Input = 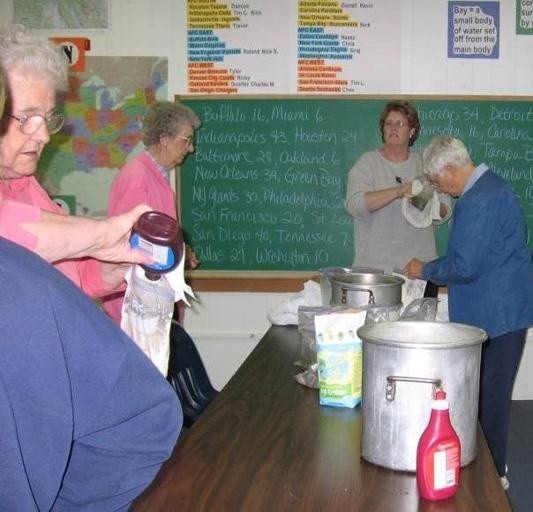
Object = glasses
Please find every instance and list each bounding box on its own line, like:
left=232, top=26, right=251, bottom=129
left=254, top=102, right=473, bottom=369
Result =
left=383, top=117, right=420, bottom=133
left=173, top=133, right=194, bottom=148
left=3, top=104, right=66, bottom=138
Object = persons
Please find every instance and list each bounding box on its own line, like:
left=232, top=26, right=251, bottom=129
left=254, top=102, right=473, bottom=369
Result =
left=0, top=61, right=183, bottom=512
left=343, top=101, right=452, bottom=298
left=107, top=99, right=201, bottom=323
left=0, top=19, right=156, bottom=299
left=404, top=134, right=533, bottom=492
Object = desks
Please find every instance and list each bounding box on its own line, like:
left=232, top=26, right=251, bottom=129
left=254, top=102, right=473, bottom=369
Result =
left=129, top=323, right=516, bottom=512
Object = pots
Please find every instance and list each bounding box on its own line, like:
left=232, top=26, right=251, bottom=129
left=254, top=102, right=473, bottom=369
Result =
left=355, top=321, right=487, bottom=473
left=315, top=266, right=405, bottom=307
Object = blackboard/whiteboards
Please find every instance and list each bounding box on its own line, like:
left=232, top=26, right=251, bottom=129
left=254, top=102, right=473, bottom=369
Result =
left=175, top=95, right=533, bottom=280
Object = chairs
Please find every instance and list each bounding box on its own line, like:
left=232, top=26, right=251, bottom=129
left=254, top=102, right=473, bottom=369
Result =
left=166, top=320, right=218, bottom=423
